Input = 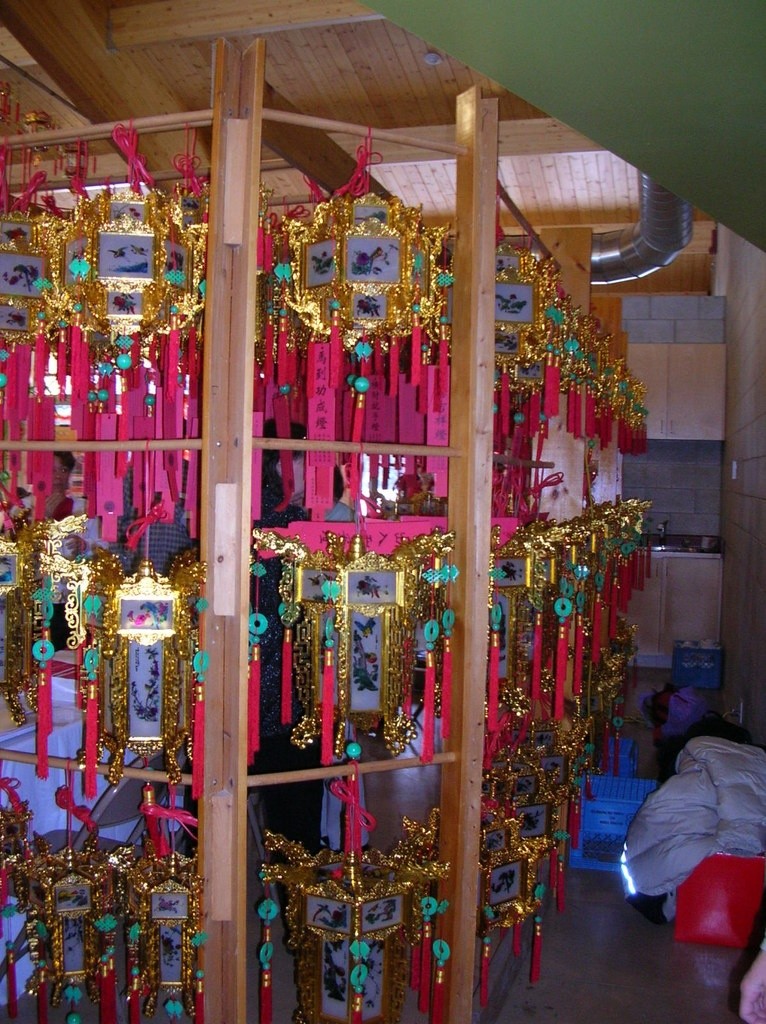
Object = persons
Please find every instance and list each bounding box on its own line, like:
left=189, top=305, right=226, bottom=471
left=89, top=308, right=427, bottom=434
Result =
left=8, top=417, right=370, bottom=955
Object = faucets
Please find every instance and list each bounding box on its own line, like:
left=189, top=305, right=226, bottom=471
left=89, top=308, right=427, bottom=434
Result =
left=656, top=520, right=668, bottom=539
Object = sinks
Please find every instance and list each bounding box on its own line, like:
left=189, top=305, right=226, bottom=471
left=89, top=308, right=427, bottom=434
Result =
left=650, top=533, right=723, bottom=553
